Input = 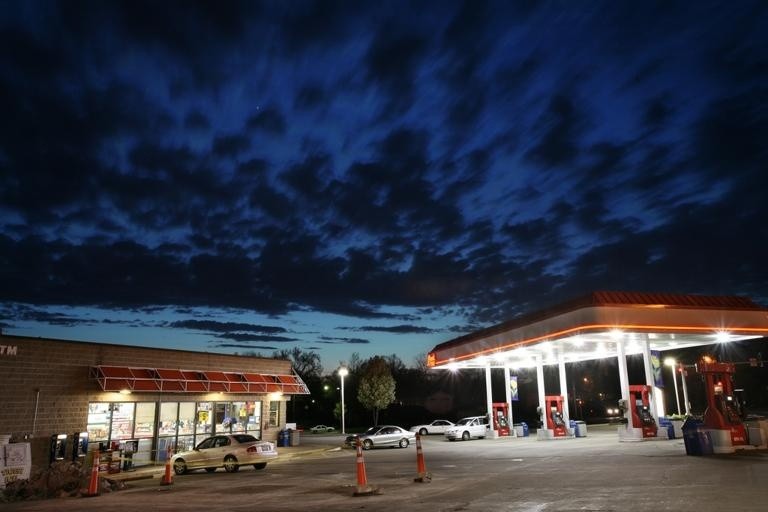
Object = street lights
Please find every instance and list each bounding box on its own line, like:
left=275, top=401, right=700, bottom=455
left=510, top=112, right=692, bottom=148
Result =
left=661, top=356, right=681, bottom=415
left=571, top=375, right=592, bottom=420
left=337, top=367, right=348, bottom=434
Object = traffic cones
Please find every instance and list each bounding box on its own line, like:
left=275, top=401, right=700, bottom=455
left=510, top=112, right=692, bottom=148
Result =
left=347, top=442, right=377, bottom=497
left=411, top=432, right=430, bottom=482
left=81, top=449, right=104, bottom=497
left=159, top=444, right=175, bottom=486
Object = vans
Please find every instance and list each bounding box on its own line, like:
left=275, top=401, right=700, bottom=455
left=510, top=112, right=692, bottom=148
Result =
left=443, top=416, right=489, bottom=443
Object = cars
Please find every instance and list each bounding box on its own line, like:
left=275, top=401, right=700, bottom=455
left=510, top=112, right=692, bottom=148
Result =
left=409, top=419, right=456, bottom=437
left=603, top=406, right=619, bottom=417
left=310, top=425, right=336, bottom=435
left=296, top=426, right=305, bottom=432
left=169, top=433, right=278, bottom=477
left=344, top=424, right=417, bottom=451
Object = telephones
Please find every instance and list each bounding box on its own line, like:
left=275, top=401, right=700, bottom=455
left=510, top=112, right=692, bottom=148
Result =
left=55, top=441, right=62, bottom=450
left=78, top=439, right=84, bottom=449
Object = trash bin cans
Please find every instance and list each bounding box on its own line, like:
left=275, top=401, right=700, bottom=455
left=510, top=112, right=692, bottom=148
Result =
left=681, top=417, right=714, bottom=456
left=278, top=430, right=299, bottom=447
left=513, top=422, right=528, bottom=437
left=659, top=417, right=685, bottom=440
left=744, top=417, right=768, bottom=445
left=569, top=420, right=587, bottom=438
